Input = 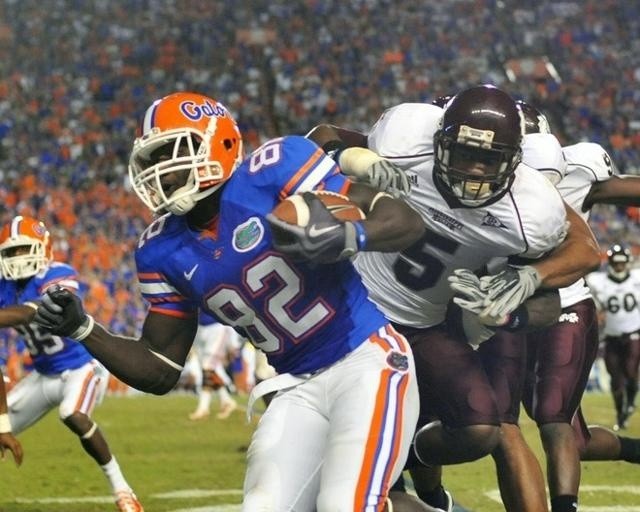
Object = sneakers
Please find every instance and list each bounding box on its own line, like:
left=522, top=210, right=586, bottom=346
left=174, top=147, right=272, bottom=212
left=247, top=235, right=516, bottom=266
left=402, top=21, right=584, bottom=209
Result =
left=187, top=408, right=209, bottom=421
left=113, top=488, right=145, bottom=511
left=217, top=399, right=236, bottom=419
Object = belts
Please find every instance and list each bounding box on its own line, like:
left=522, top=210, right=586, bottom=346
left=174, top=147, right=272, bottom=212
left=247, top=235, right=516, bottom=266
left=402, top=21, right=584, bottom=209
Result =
left=246, top=358, right=315, bottom=425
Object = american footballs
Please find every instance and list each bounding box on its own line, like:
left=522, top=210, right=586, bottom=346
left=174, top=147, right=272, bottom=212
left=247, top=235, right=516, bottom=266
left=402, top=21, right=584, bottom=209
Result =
left=272, top=190, right=365, bottom=228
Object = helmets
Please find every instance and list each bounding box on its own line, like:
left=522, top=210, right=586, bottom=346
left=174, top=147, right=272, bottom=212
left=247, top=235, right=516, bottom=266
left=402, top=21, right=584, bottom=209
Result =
left=605, top=243, right=634, bottom=282
left=127, top=92, right=242, bottom=219
left=1, top=215, right=53, bottom=283
left=431, top=83, right=524, bottom=210
left=518, top=98, right=553, bottom=137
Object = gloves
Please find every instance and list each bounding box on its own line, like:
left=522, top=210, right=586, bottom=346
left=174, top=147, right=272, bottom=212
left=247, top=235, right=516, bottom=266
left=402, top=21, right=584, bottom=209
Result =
left=263, top=192, right=360, bottom=268
left=32, top=284, right=96, bottom=344
left=449, top=267, right=508, bottom=328
left=477, top=264, right=543, bottom=319
left=367, top=159, right=412, bottom=199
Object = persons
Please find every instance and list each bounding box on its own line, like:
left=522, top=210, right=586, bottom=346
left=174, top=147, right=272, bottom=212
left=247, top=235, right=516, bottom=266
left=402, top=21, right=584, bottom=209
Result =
left=0, top=0, right=639, bottom=511
